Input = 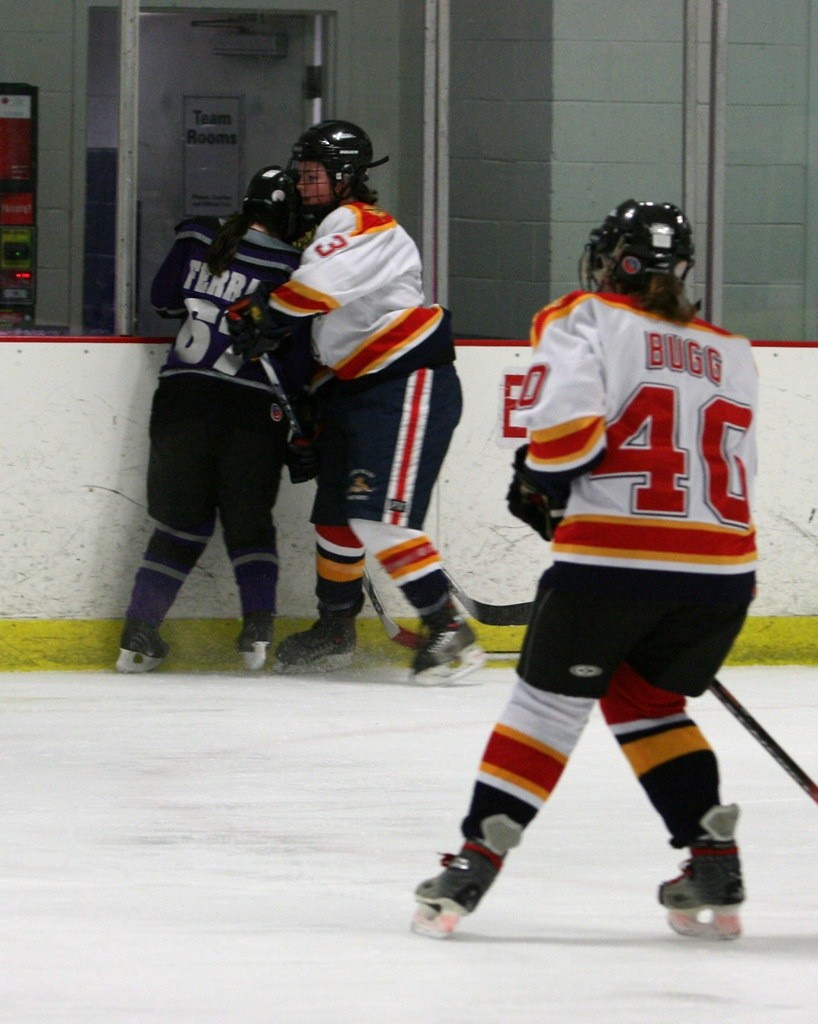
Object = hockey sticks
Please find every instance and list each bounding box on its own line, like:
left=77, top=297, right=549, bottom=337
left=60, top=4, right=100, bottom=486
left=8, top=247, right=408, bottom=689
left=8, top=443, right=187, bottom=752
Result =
left=436, top=561, right=536, bottom=627
left=261, top=350, right=435, bottom=651
left=554, top=517, right=818, bottom=805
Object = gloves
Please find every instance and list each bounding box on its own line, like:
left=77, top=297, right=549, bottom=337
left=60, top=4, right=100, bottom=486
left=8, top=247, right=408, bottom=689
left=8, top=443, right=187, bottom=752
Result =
left=283, top=417, right=332, bottom=484
left=223, top=292, right=286, bottom=361
left=504, top=443, right=575, bottom=541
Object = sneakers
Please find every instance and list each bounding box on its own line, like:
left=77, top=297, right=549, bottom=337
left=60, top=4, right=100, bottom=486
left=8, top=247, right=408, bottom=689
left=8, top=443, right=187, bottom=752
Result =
left=657, top=803, right=746, bottom=940
left=408, top=598, right=484, bottom=687
left=271, top=592, right=366, bottom=675
left=235, top=609, right=276, bottom=671
left=115, top=615, right=170, bottom=673
left=409, top=815, right=521, bottom=938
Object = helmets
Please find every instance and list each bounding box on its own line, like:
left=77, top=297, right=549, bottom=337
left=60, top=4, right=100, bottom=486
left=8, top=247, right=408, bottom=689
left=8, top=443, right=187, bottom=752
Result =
left=577, top=199, right=697, bottom=300
left=242, top=164, right=318, bottom=251
left=283, top=118, right=374, bottom=218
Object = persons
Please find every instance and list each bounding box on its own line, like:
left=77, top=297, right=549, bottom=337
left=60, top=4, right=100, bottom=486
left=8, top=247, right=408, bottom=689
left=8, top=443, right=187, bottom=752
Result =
left=409, top=200, right=760, bottom=940
left=115, top=165, right=313, bottom=674
left=224, top=119, right=486, bottom=685
